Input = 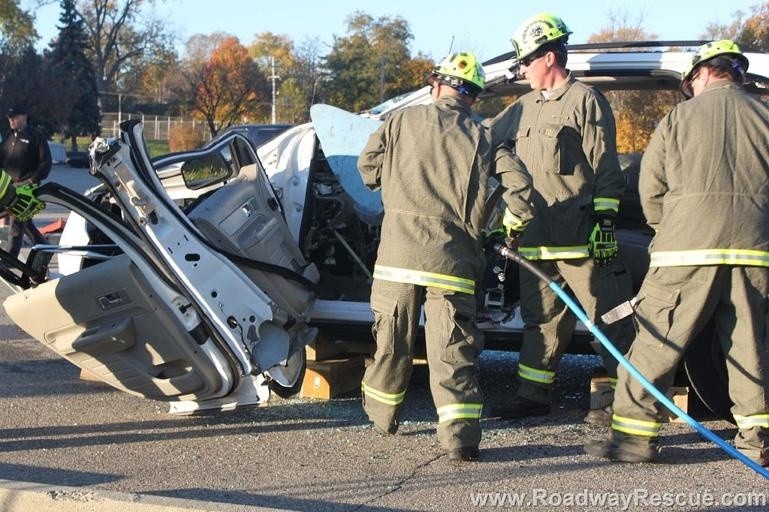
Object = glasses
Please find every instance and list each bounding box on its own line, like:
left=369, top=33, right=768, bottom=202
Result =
left=519, top=47, right=542, bottom=66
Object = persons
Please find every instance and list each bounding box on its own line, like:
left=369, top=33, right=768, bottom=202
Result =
left=583, top=40, right=769, bottom=468
left=0, top=167, right=46, bottom=224
left=487, top=14, right=640, bottom=427
left=355, top=52, right=536, bottom=462
left=0, top=105, right=52, bottom=267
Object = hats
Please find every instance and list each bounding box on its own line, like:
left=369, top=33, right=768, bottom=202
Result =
left=5, top=104, right=30, bottom=116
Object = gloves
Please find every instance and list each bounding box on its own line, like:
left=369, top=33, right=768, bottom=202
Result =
left=7, top=183, right=46, bottom=223
left=485, top=226, right=522, bottom=244
left=585, top=215, right=618, bottom=268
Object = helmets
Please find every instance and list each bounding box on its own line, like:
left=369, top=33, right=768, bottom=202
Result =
left=679, top=39, right=749, bottom=98
left=426, top=51, right=486, bottom=90
left=513, top=12, right=573, bottom=63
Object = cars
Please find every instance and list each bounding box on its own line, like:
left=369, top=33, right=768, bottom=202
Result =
left=0, top=35, right=769, bottom=417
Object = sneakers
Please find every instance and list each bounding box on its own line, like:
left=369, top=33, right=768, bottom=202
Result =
left=489, top=393, right=550, bottom=417
left=749, top=455, right=769, bottom=466
left=372, top=417, right=398, bottom=435
left=449, top=446, right=479, bottom=459
left=583, top=404, right=613, bottom=425
left=584, top=439, right=650, bottom=463
